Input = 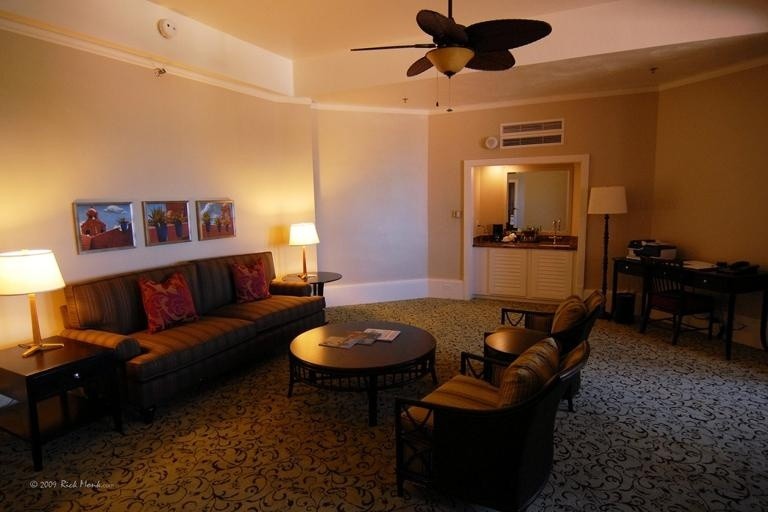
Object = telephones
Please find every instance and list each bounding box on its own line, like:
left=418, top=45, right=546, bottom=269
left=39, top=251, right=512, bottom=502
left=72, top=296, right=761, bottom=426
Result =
left=730, top=261, right=760, bottom=272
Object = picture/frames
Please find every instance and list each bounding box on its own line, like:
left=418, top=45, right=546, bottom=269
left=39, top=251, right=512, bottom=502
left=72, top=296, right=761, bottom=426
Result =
left=195, top=200, right=236, bottom=241
left=72, top=201, right=138, bottom=255
left=142, top=200, right=192, bottom=247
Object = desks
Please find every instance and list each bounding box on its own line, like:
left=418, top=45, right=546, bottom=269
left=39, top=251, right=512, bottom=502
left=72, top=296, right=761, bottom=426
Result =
left=611, top=257, right=768, bottom=360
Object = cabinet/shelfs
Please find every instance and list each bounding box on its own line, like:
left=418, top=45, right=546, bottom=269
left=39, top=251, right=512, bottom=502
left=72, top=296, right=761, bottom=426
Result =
left=0, top=336, right=124, bottom=472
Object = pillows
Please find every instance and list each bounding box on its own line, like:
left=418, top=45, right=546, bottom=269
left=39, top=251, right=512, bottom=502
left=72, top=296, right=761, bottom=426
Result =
left=496, top=337, right=559, bottom=409
left=232, top=257, right=271, bottom=304
left=137, top=272, right=200, bottom=335
left=551, top=295, right=587, bottom=334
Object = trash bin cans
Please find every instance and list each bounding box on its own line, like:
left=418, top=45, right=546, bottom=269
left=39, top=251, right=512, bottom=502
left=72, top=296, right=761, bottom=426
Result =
left=614, top=292, right=635, bottom=324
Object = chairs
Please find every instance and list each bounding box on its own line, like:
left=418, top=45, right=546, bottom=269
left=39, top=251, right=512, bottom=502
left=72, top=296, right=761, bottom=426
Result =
left=395, top=336, right=561, bottom=512
left=640, top=257, right=716, bottom=346
left=484, top=289, right=607, bottom=413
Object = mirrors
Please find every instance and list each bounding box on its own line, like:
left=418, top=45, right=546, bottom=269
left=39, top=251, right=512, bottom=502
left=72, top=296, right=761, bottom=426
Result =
left=476, top=167, right=572, bottom=237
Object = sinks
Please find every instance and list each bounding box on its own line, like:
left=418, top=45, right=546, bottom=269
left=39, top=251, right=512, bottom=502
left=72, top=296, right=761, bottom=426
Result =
left=537, top=244, right=570, bottom=247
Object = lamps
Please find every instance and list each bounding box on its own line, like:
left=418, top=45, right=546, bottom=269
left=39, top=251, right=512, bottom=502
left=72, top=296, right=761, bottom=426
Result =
left=289, top=222, right=320, bottom=275
left=588, top=186, right=628, bottom=318
left=426, top=48, right=474, bottom=113
left=1, top=248, right=66, bottom=358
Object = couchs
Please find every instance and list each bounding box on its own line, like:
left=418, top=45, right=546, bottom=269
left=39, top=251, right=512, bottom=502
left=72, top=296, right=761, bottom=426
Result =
left=61, top=251, right=326, bottom=436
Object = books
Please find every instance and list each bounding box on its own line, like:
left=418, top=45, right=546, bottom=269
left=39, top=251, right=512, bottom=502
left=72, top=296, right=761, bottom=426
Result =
left=318, top=328, right=400, bottom=349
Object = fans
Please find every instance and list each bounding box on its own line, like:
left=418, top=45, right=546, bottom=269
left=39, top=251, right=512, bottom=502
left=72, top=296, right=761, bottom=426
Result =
left=351, top=1, right=552, bottom=78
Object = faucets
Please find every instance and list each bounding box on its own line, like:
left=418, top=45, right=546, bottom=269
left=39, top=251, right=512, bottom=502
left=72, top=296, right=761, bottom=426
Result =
left=552, top=219, right=557, bottom=236
left=557, top=218, right=561, bottom=233
